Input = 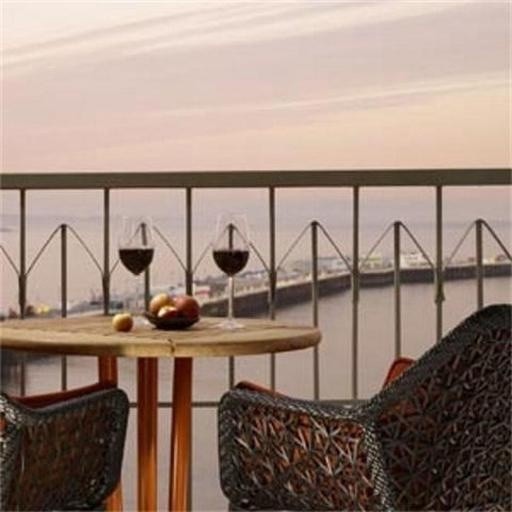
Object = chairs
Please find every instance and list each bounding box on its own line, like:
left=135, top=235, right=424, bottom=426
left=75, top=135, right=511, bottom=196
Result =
left=214, top=299, right=510, bottom=511
left=1, top=379, right=130, bottom=511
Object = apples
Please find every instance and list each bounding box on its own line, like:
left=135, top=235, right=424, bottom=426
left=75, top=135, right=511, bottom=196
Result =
left=113, top=314, right=133, bottom=331
left=150, top=294, right=201, bottom=318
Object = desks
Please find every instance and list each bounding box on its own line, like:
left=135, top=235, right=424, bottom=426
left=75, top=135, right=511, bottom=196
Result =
left=1, top=311, right=325, bottom=512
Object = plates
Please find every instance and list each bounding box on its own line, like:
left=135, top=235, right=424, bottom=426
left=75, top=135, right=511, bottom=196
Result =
left=147, top=313, right=199, bottom=330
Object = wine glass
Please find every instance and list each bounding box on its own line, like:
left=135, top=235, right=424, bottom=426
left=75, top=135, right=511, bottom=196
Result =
left=119, top=215, right=155, bottom=328
left=213, top=214, right=250, bottom=328
